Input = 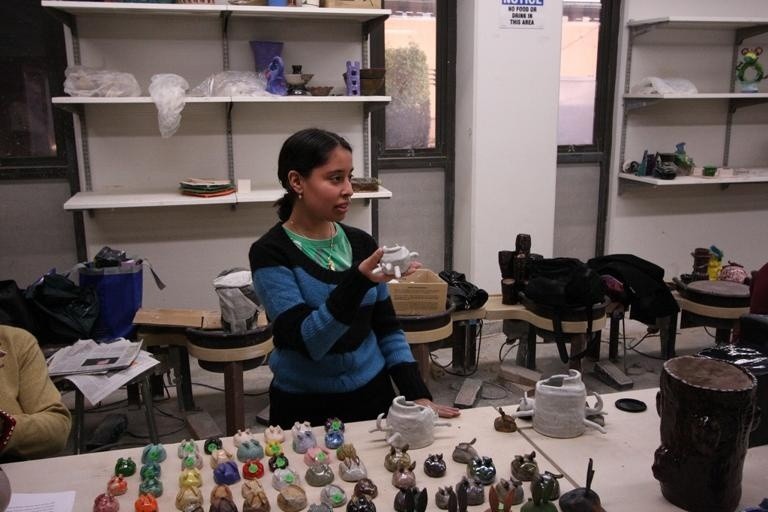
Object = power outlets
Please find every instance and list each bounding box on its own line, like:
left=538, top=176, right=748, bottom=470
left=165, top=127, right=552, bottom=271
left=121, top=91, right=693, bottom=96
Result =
left=430, top=358, right=446, bottom=380
left=642, top=322, right=663, bottom=335
left=535, top=333, right=544, bottom=343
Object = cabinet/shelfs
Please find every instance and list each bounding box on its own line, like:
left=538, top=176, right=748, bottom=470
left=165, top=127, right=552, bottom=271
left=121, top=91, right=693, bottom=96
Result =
left=41, top=1, right=393, bottom=306
left=595, top=0, right=768, bottom=282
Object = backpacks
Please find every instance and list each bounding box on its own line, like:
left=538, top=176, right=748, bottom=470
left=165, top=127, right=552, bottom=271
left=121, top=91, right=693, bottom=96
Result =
left=26, top=270, right=104, bottom=341
left=518, top=256, right=605, bottom=363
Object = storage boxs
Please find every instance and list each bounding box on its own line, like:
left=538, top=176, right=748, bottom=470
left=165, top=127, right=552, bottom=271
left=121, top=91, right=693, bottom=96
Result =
left=387, top=266, right=447, bottom=316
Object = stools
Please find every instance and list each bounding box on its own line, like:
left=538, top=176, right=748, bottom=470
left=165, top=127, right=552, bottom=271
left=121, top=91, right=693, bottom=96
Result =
left=56, top=350, right=171, bottom=454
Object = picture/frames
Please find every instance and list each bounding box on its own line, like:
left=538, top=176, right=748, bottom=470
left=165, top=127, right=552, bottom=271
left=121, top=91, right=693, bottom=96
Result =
left=0, top=2, right=77, bottom=180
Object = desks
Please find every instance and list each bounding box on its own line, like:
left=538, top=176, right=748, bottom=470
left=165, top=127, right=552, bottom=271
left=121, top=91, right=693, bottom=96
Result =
left=496, top=388, right=768, bottom=512
left=0, top=406, right=589, bottom=512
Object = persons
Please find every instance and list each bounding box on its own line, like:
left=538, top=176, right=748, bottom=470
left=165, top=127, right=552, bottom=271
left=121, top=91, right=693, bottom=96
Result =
left=250, top=128, right=460, bottom=433
left=1, top=324, right=72, bottom=463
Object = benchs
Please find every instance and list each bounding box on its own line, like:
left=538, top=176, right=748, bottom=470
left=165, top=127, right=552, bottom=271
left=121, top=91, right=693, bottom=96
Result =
left=133, top=310, right=487, bottom=434
left=486, top=282, right=750, bottom=381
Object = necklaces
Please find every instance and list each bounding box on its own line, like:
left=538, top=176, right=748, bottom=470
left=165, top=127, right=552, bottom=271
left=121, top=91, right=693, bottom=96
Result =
left=288, top=218, right=336, bottom=272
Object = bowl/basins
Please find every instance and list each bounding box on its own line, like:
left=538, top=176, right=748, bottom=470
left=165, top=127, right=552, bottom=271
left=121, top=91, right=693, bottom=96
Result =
left=341, top=69, right=385, bottom=78
left=310, top=84, right=334, bottom=97
left=282, top=73, right=315, bottom=88
left=342, top=78, right=388, bottom=96
